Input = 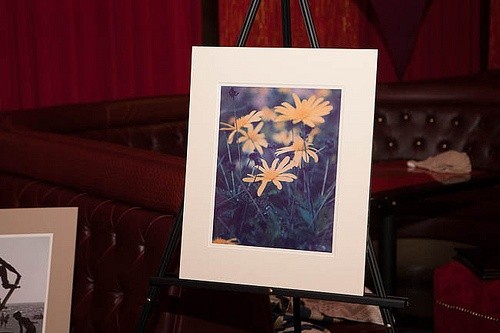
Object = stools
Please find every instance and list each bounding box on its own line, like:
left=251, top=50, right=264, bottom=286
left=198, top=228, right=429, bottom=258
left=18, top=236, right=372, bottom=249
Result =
left=435, top=261, right=500, bottom=333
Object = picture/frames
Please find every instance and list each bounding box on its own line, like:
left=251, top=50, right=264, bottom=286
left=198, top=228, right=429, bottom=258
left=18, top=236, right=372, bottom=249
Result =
left=0, top=206, right=79, bottom=333
left=178, top=44, right=379, bottom=297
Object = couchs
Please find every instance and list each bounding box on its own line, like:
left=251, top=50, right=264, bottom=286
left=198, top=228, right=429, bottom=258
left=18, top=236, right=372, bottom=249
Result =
left=0, top=75, right=500, bottom=332
